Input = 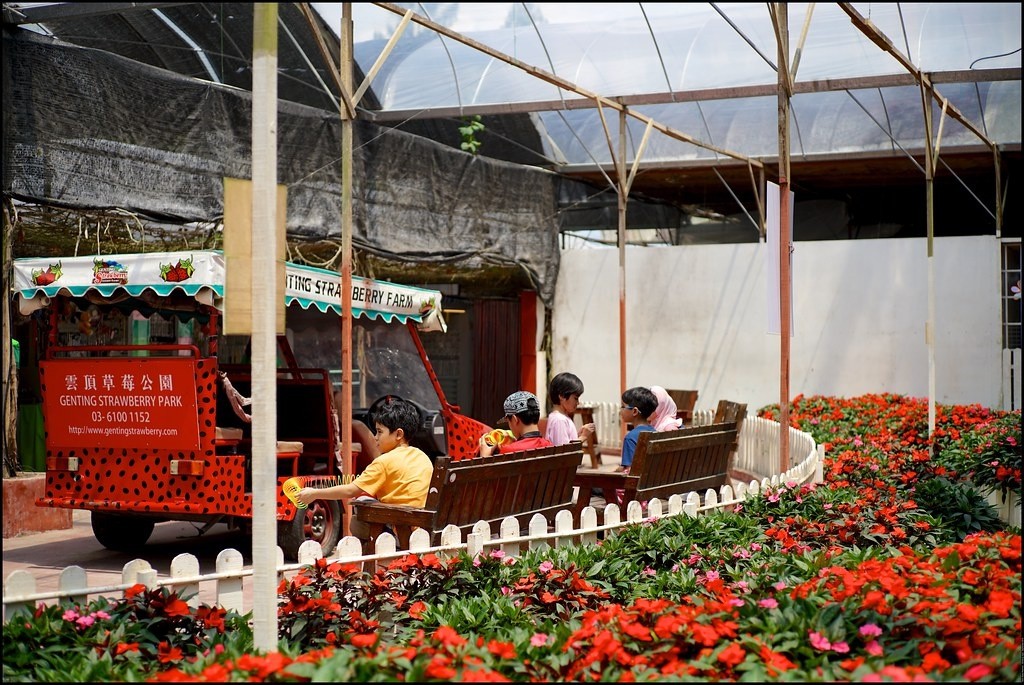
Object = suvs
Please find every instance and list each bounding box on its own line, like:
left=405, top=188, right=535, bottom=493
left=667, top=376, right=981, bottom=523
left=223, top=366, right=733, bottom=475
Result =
left=13, top=253, right=494, bottom=560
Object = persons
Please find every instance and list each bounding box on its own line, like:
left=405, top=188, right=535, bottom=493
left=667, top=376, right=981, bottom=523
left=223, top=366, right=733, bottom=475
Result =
left=479, top=391, right=553, bottom=458
left=544, top=373, right=594, bottom=446
left=647, top=386, right=683, bottom=432
left=295, top=402, right=436, bottom=535
left=588, top=386, right=658, bottom=496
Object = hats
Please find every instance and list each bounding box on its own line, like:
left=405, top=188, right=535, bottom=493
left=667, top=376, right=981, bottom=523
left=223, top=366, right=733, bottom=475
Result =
left=497, top=391, right=541, bottom=425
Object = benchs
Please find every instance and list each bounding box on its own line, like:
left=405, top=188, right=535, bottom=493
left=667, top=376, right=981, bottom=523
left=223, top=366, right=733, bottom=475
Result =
left=574, top=420, right=737, bottom=526
left=353, top=439, right=589, bottom=551
left=668, top=387, right=747, bottom=456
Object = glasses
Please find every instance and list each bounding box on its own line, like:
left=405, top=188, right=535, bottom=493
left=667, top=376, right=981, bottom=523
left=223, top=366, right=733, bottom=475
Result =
left=620, top=405, right=642, bottom=414
